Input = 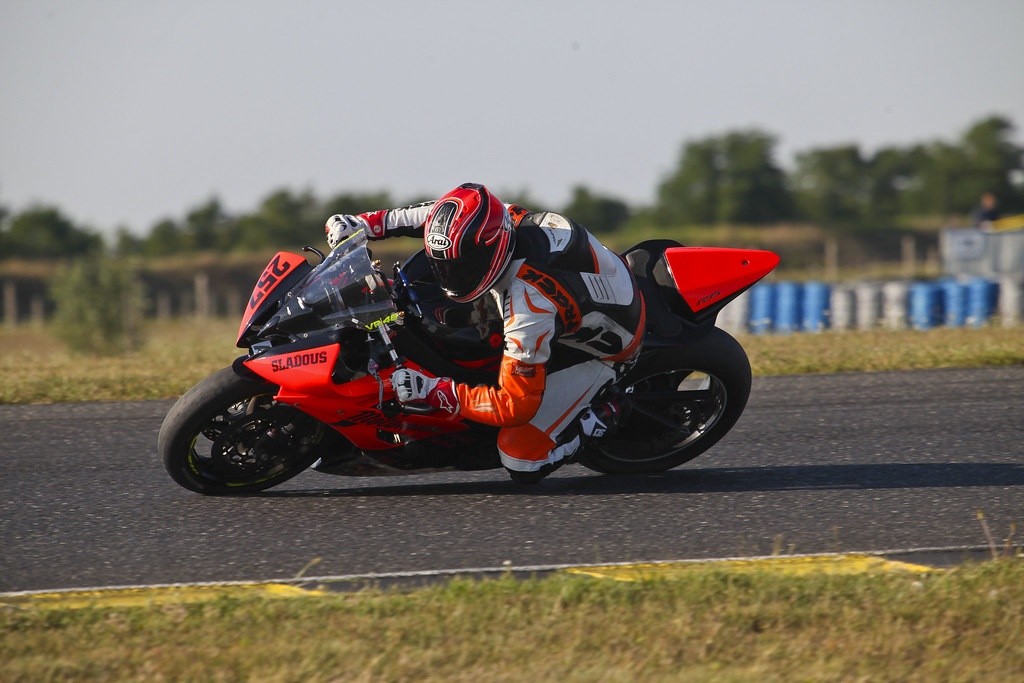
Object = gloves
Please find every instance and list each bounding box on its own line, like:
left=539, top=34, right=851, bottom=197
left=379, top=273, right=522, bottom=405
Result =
left=389, top=368, right=457, bottom=422
left=321, top=210, right=387, bottom=252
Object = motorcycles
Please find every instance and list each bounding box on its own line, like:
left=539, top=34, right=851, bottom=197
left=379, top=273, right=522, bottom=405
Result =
left=156, top=226, right=780, bottom=497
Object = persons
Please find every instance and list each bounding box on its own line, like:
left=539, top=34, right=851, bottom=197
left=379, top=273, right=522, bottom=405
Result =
left=324, top=182, right=646, bottom=487
left=972, top=191, right=1000, bottom=229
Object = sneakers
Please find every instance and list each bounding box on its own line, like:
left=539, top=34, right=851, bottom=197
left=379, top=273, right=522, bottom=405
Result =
left=578, top=394, right=627, bottom=450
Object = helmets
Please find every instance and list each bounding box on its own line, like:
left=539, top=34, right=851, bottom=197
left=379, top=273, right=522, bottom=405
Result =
left=421, top=183, right=513, bottom=305
